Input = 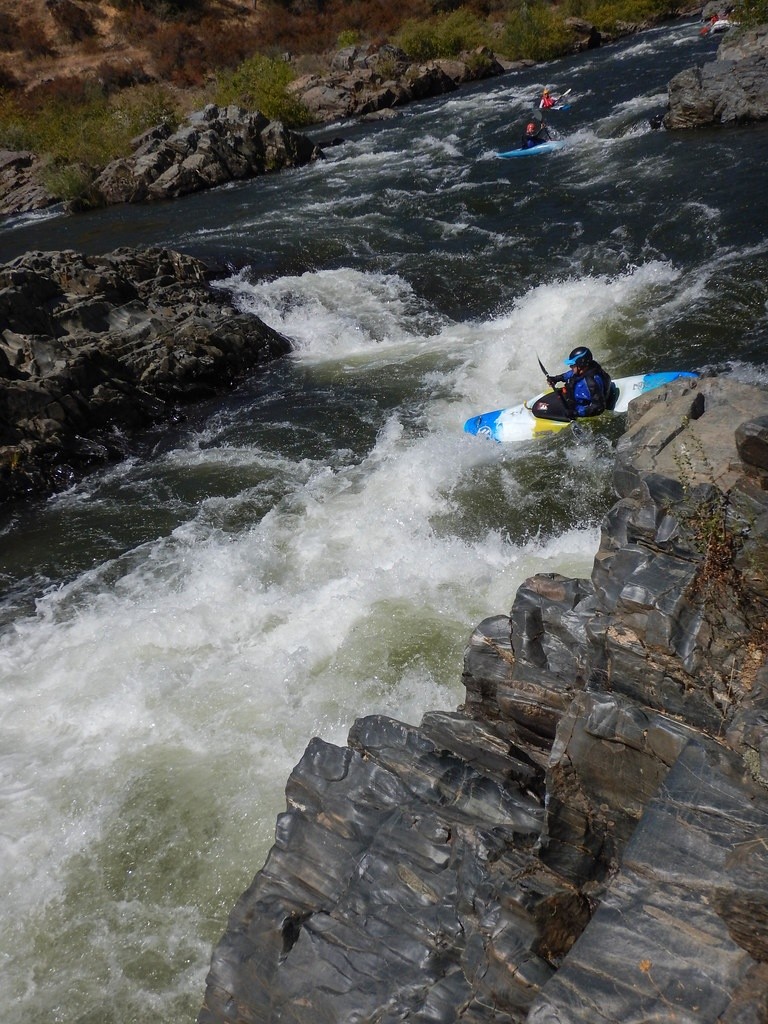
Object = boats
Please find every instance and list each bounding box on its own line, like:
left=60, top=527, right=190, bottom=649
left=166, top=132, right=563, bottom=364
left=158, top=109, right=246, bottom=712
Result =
left=550, top=104, right=574, bottom=113
left=497, top=139, right=564, bottom=158
left=463, top=370, right=697, bottom=444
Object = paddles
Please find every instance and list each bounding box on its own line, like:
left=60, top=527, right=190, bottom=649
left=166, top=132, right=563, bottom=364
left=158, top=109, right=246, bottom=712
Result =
left=536, top=351, right=579, bottom=421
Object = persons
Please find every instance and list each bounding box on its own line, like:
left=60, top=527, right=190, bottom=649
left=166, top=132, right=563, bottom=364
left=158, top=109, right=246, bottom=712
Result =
left=539, top=346, right=616, bottom=419
left=521, top=123, right=548, bottom=151
left=710, top=13, right=720, bottom=26
left=539, top=90, right=564, bottom=109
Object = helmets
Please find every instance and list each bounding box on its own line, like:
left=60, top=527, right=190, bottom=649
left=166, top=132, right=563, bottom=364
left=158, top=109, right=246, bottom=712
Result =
left=564, top=347, right=592, bottom=368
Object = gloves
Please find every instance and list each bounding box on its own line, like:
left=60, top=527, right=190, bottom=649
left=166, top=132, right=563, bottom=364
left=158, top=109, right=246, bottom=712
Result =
left=546, top=376, right=557, bottom=386
left=564, top=407, right=578, bottom=417
